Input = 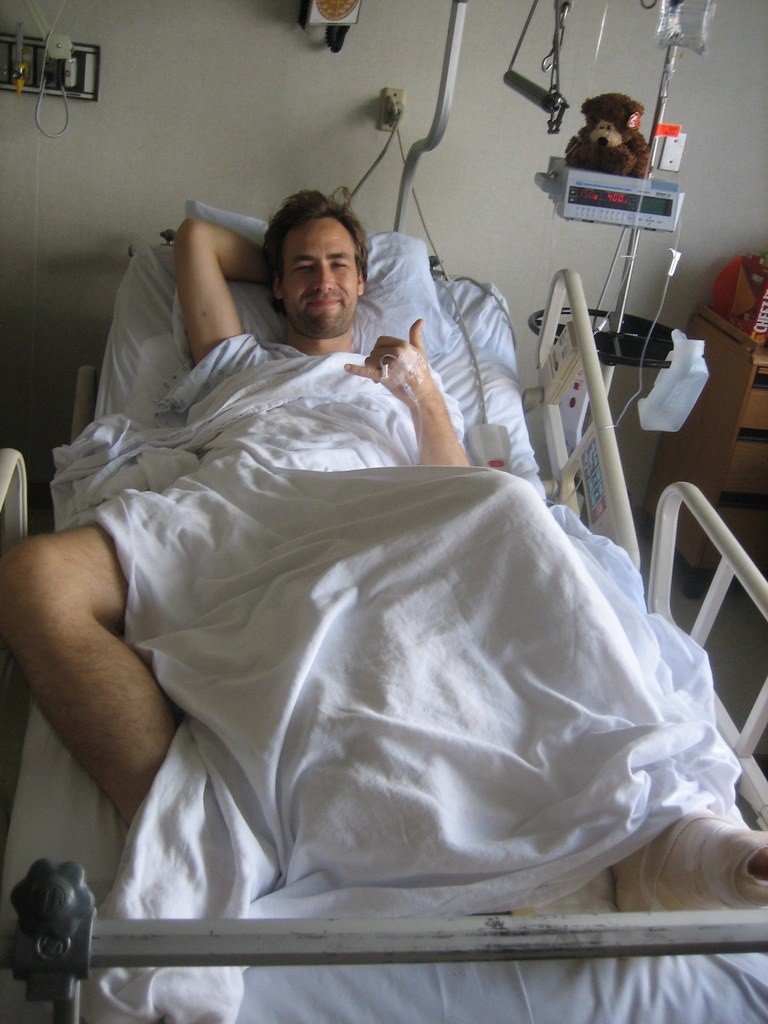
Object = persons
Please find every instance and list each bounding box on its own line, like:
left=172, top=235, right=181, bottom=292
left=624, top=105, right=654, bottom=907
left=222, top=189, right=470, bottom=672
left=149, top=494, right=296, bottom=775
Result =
left=0, top=190, right=768, bottom=915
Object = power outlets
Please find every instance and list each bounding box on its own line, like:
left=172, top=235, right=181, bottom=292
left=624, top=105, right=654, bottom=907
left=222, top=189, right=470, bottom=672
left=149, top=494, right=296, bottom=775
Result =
left=377, top=86, right=405, bottom=132
left=36, top=47, right=59, bottom=91
left=173, top=199, right=462, bottom=372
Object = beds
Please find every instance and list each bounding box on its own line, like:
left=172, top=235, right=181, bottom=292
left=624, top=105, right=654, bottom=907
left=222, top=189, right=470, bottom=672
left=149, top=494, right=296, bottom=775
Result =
left=0, top=241, right=768, bottom=1024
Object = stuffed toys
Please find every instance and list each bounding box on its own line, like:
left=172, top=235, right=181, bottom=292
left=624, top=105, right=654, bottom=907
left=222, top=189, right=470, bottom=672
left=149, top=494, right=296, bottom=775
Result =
left=565, top=94, right=654, bottom=179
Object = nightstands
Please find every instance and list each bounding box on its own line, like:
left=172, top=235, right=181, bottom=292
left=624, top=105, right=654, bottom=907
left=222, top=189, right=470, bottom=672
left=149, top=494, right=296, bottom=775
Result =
left=641, top=302, right=768, bottom=600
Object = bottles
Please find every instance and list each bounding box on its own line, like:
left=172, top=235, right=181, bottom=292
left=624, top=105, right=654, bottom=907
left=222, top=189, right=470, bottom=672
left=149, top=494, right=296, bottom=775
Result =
left=638, top=339, right=709, bottom=432
